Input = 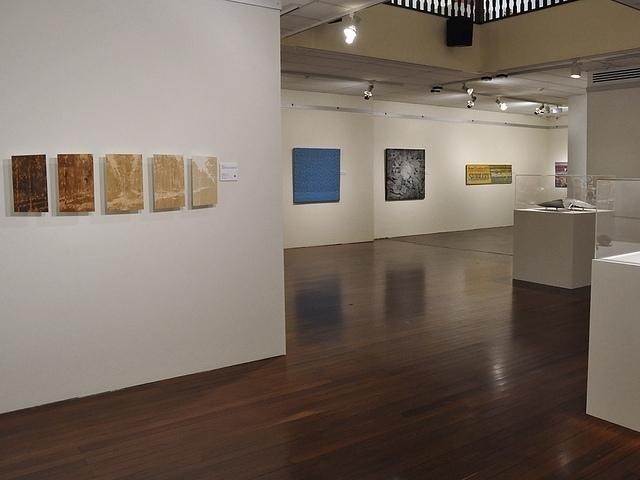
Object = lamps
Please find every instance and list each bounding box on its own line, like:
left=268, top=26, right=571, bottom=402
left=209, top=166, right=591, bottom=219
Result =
left=570, top=58, right=581, bottom=79
left=461, top=83, right=562, bottom=115
left=344, top=14, right=362, bottom=45
left=363, top=85, right=374, bottom=100
